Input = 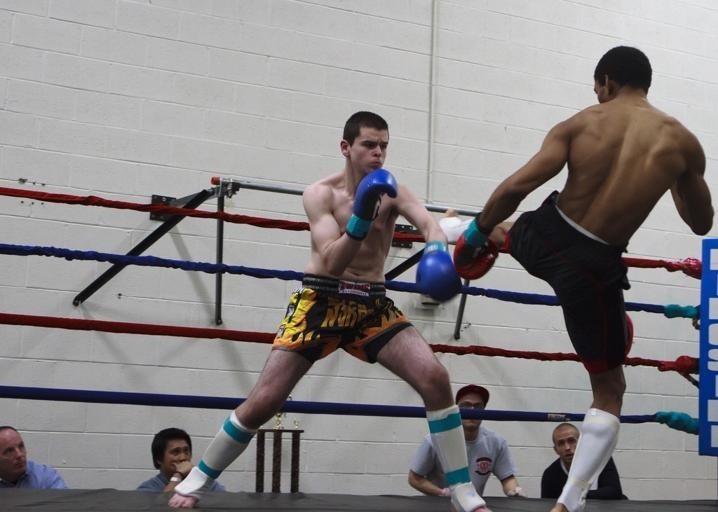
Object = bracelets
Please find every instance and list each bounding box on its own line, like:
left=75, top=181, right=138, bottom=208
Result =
left=172, top=470, right=185, bottom=478
left=169, top=477, right=181, bottom=482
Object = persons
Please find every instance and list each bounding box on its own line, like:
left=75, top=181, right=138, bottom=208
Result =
left=437, top=43, right=715, bottom=511
left=133, top=426, right=226, bottom=491
left=0, top=426, right=66, bottom=490
left=541, top=422, right=629, bottom=500
left=408, top=382, right=527, bottom=498
left=169, top=111, right=496, bottom=510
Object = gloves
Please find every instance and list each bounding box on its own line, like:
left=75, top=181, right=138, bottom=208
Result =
left=346, top=169, right=397, bottom=241
left=416, top=241, right=462, bottom=302
left=439, top=488, right=451, bottom=497
left=453, top=217, right=498, bottom=280
left=507, top=487, right=526, bottom=498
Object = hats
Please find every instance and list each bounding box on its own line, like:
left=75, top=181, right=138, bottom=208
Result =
left=456, top=384, right=489, bottom=408
